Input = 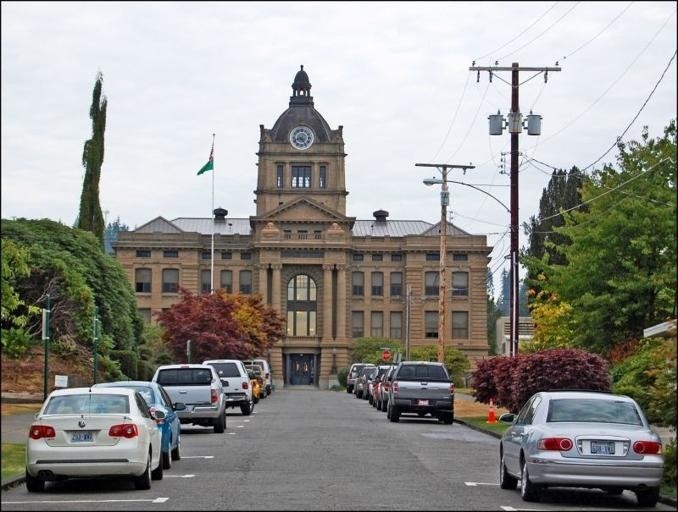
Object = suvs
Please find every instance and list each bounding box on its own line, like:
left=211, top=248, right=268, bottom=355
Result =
left=345, top=360, right=455, bottom=425
left=152, top=365, right=226, bottom=433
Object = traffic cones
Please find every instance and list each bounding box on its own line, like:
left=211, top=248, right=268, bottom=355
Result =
left=485, top=397, right=497, bottom=425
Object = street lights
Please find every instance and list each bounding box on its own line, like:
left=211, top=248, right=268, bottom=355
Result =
left=419, top=178, right=516, bottom=367
left=329, top=347, right=337, bottom=376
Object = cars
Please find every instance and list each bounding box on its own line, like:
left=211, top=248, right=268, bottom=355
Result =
left=499, top=392, right=665, bottom=512
left=82, top=381, right=186, bottom=470
left=25, top=386, right=164, bottom=492
left=203, top=357, right=272, bottom=415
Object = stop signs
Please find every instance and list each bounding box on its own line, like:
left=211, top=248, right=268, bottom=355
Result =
left=380, top=351, right=391, bottom=361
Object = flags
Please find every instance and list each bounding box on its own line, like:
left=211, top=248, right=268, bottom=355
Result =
left=195, top=136, right=214, bottom=176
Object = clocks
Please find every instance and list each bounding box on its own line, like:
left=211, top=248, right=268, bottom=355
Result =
left=286, top=124, right=317, bottom=153
